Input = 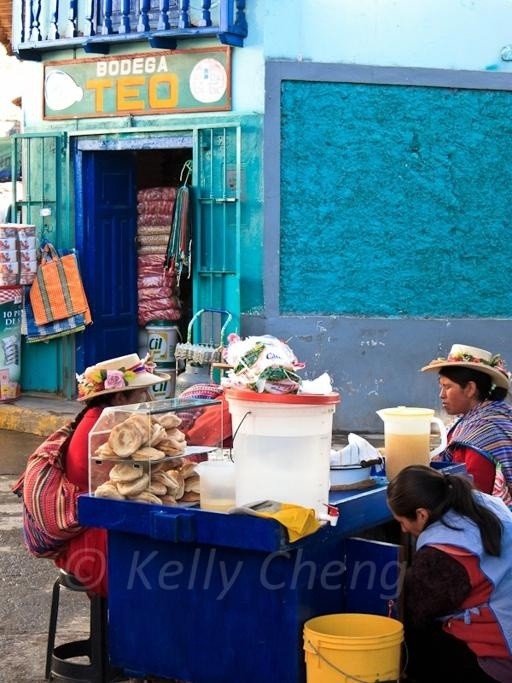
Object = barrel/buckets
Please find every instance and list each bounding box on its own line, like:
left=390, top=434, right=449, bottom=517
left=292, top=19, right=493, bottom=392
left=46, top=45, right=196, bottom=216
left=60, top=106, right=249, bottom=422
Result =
left=302, top=614, right=410, bottom=682
left=148, top=366, right=176, bottom=400
left=145, top=319, right=183, bottom=366
left=225, top=384, right=340, bottom=528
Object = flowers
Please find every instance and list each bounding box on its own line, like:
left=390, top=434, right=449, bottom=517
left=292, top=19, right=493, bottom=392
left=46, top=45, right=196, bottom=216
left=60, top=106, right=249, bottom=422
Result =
left=431, top=351, right=512, bottom=380
left=75, top=354, right=157, bottom=399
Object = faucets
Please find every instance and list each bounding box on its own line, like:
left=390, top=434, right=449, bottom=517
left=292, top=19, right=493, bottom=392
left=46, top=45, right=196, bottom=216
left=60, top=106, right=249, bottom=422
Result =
left=315, top=503, right=340, bottom=529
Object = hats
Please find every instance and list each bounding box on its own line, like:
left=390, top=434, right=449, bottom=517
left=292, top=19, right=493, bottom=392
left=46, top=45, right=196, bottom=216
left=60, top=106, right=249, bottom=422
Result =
left=419, top=343, right=511, bottom=390
left=77, top=352, right=172, bottom=401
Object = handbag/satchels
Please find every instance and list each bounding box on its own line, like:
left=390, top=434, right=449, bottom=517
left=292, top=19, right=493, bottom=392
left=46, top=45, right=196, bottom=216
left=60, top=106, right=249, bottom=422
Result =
left=176, top=384, right=223, bottom=439
left=20, top=239, right=94, bottom=344
left=13, top=417, right=89, bottom=556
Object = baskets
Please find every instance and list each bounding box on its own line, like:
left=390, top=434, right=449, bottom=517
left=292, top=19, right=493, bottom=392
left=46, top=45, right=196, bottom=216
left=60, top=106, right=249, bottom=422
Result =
left=174, top=308, right=232, bottom=364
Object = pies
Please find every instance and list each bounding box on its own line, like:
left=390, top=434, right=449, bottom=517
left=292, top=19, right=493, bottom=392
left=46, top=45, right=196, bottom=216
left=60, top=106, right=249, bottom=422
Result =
left=94, top=408, right=202, bottom=506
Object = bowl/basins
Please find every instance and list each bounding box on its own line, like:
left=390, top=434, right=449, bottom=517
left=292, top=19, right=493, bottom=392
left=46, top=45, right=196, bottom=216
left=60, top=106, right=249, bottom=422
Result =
left=330, top=456, right=382, bottom=487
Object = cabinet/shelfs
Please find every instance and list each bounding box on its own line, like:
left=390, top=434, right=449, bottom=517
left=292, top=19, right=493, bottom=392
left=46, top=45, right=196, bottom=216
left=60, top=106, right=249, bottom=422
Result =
left=87, top=398, right=224, bottom=507
left=77, top=463, right=470, bottom=682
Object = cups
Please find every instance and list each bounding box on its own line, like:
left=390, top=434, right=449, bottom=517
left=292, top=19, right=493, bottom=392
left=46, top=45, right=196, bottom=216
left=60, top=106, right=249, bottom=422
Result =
left=194, top=460, right=237, bottom=514
left=376, top=405, right=449, bottom=483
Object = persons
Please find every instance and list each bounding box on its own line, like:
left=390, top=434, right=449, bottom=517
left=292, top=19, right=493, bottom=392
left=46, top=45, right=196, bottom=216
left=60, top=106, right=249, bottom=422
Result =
left=11, top=353, right=171, bottom=599
left=419, top=341, right=511, bottom=511
left=388, top=464, right=512, bottom=682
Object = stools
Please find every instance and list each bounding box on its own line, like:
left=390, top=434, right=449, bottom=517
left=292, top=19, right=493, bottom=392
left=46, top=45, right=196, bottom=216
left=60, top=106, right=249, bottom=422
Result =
left=45, top=569, right=107, bottom=683
